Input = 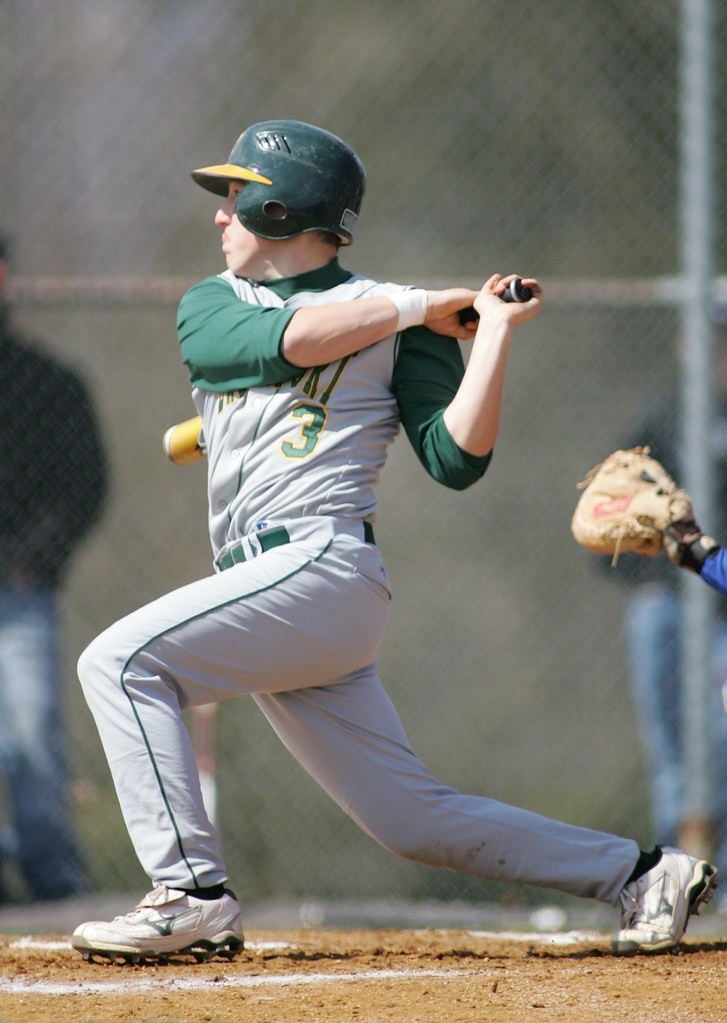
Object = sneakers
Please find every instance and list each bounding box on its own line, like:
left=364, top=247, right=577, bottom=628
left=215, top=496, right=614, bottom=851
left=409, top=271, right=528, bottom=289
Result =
left=616, top=844, right=717, bottom=953
left=70, top=887, right=245, bottom=965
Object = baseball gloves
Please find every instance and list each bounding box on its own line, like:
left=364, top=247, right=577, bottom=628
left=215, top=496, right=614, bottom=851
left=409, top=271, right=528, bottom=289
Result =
left=571, top=449, right=702, bottom=555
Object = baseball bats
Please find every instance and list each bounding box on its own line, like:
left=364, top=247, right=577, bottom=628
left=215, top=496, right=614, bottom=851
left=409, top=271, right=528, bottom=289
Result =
left=161, top=277, right=531, bottom=464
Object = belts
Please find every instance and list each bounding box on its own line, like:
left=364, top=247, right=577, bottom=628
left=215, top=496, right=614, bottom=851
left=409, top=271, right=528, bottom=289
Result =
left=217, top=520, right=374, bottom=571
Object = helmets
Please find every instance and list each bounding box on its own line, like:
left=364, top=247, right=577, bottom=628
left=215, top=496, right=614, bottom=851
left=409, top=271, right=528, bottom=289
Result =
left=191, top=119, right=366, bottom=243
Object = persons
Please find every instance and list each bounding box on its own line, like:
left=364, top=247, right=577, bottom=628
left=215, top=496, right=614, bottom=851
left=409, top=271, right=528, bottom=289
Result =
left=573, top=275, right=726, bottom=919
left=0, top=229, right=107, bottom=902
left=73, top=121, right=721, bottom=963
